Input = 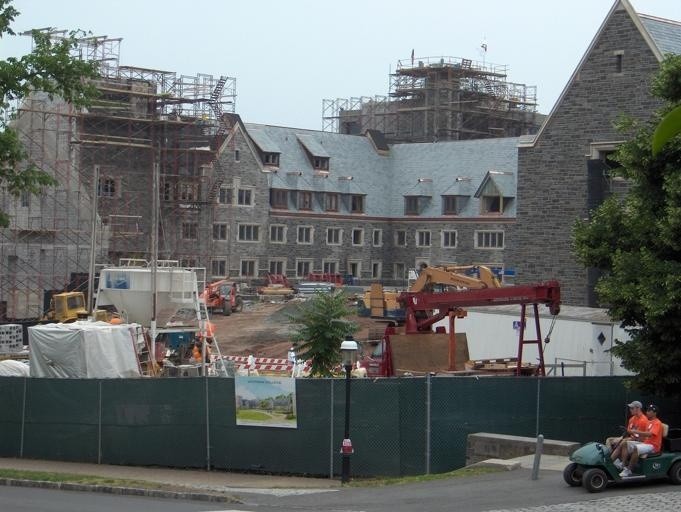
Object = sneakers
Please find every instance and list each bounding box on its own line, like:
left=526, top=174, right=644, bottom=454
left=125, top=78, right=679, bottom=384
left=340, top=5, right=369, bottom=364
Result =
left=619, top=468, right=633, bottom=478
left=612, top=458, right=626, bottom=471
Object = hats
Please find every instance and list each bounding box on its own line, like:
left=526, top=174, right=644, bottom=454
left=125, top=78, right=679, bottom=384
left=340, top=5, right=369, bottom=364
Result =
left=646, top=404, right=658, bottom=413
left=626, top=400, right=643, bottom=411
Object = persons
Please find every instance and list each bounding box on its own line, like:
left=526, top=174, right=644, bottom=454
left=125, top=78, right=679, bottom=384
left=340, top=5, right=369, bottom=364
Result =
left=288, top=343, right=298, bottom=364
left=614, top=404, right=663, bottom=477
left=606, top=401, right=649, bottom=463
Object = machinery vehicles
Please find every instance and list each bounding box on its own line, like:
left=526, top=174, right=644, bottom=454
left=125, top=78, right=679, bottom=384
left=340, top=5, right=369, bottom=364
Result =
left=39, top=291, right=90, bottom=323
left=357, top=260, right=501, bottom=329
left=200, top=273, right=244, bottom=314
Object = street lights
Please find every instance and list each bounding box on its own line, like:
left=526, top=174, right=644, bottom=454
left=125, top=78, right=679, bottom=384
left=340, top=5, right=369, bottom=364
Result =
left=339, top=334, right=359, bottom=485
